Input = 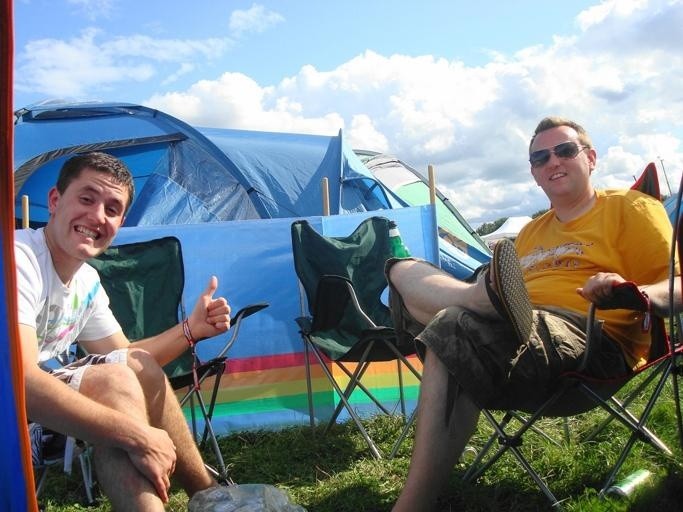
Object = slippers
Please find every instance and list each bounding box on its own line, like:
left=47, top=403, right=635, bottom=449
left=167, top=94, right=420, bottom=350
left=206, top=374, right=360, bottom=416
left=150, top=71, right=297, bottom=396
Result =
left=485, top=238, right=533, bottom=346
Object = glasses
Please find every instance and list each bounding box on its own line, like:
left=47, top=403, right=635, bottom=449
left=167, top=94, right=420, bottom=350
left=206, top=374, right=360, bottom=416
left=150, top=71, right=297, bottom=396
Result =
left=530, top=140, right=589, bottom=168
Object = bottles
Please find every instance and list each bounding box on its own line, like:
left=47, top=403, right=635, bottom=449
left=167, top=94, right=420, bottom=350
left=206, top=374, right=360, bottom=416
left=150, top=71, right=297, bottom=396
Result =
left=601, top=470, right=651, bottom=504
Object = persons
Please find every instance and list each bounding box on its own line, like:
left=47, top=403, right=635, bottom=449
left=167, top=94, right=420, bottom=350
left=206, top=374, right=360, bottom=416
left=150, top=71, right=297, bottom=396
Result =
left=385, top=116, right=677, bottom=510
left=15, top=150, right=231, bottom=511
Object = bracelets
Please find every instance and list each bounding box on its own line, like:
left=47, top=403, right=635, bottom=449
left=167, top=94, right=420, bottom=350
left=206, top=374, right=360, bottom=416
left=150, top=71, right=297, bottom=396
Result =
left=181, top=318, right=196, bottom=348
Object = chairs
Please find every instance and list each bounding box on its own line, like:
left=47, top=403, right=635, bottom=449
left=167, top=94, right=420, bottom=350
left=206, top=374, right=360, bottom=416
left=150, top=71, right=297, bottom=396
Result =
left=462, top=163, right=683, bottom=512
left=35, top=236, right=270, bottom=511
left=291, top=216, right=422, bottom=461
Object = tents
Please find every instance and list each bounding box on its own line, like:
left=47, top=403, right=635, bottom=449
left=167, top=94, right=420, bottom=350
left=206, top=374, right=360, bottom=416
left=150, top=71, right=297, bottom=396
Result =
left=15, top=98, right=391, bottom=224
left=477, top=216, right=534, bottom=242
left=353, top=150, right=491, bottom=256
